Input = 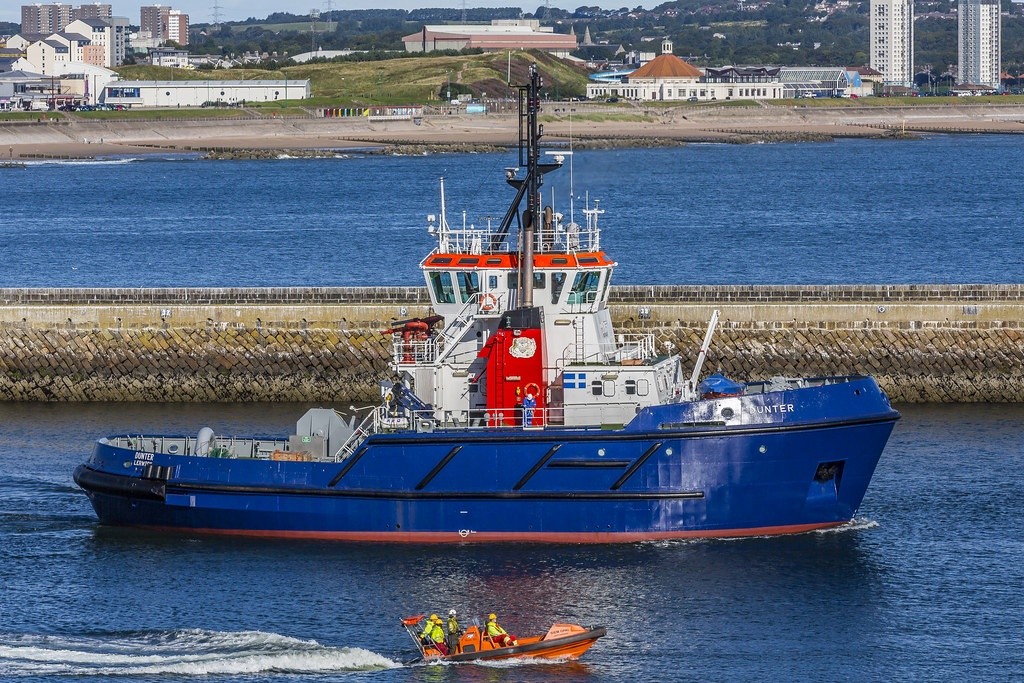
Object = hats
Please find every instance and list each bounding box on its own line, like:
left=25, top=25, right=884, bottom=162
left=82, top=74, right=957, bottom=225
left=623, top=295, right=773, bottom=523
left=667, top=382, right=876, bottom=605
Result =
left=436, top=619, right=443, bottom=624
left=488, top=614, right=497, bottom=619
left=527, top=394, right=533, bottom=400
left=430, top=614, right=438, bottom=619
left=449, top=610, right=456, bottom=615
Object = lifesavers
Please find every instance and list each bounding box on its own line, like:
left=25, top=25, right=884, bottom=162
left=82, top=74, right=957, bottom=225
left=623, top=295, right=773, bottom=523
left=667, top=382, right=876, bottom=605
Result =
left=479, top=293, right=497, bottom=310
left=523, top=382, right=540, bottom=398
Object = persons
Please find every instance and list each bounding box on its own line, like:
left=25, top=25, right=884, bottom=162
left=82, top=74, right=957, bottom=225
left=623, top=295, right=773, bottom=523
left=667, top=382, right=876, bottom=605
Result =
left=487, top=614, right=517, bottom=646
left=431, top=619, right=448, bottom=656
left=446, top=609, right=465, bottom=655
left=418, top=614, right=438, bottom=643
left=523, top=393, right=536, bottom=427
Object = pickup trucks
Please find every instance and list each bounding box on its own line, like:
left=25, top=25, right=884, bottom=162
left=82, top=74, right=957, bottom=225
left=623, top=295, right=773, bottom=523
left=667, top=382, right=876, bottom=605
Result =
left=802, top=91, right=817, bottom=99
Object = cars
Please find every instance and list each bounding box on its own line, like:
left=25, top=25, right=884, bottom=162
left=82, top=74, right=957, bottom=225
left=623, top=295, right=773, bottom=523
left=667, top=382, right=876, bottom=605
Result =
left=58, top=101, right=127, bottom=113
left=606, top=98, right=618, bottom=103
left=840, top=91, right=1024, bottom=99
left=687, top=97, right=699, bottom=102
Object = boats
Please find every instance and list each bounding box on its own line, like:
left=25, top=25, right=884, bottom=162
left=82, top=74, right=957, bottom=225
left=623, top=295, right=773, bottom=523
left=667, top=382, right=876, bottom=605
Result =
left=395, top=610, right=609, bottom=669
left=72, top=66, right=904, bottom=546
